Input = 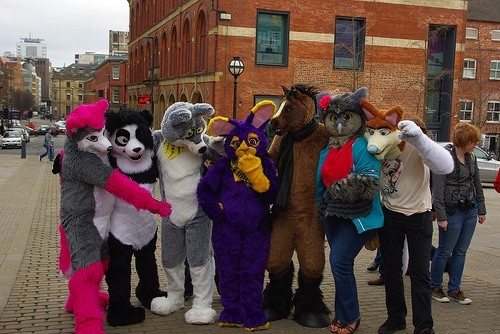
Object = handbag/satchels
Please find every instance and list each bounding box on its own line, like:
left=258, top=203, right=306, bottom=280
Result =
left=43, top=144, right=48, bottom=149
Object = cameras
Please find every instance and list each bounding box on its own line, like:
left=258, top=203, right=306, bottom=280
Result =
left=458, top=200, right=476, bottom=210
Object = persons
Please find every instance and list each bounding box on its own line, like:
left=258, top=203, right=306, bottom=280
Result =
left=431, top=123, right=486, bottom=305
left=39, top=127, right=54, bottom=162
left=366, top=168, right=438, bottom=286
left=494, top=168, right=500, bottom=193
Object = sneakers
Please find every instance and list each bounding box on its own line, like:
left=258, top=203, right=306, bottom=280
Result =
left=447, top=290, right=473, bottom=305
left=432, top=289, right=451, bottom=302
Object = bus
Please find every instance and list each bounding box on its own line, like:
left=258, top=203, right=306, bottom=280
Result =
left=2, top=110, right=28, bottom=119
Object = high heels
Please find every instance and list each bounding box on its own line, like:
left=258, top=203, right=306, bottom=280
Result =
left=330, top=316, right=360, bottom=334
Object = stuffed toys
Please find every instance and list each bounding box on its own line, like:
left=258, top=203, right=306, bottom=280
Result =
left=51, top=84, right=455, bottom=334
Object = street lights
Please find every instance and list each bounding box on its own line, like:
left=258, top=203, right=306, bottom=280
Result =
left=143, top=36, right=154, bottom=113
left=228, top=57, right=245, bottom=119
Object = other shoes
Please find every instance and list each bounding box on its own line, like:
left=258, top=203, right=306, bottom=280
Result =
left=40, top=155, right=42, bottom=161
left=414, top=327, right=435, bottom=334
left=367, top=261, right=380, bottom=271
left=368, top=277, right=385, bottom=286
left=49, top=160, right=54, bottom=162
left=378, top=319, right=406, bottom=334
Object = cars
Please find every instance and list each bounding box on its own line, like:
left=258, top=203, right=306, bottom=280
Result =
left=33, top=127, right=60, bottom=136
left=434, top=141, right=500, bottom=184
left=55, top=121, right=67, bottom=132
left=9, top=120, right=20, bottom=126
left=40, top=115, right=55, bottom=120
left=7, top=128, right=30, bottom=143
left=1, top=131, right=22, bottom=149
left=13, top=126, right=34, bottom=135
